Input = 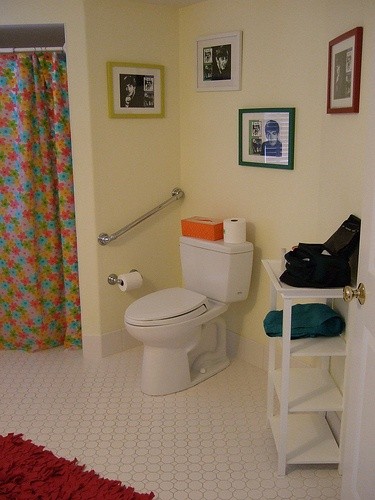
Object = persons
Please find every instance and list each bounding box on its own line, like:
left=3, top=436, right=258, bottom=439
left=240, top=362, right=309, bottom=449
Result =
left=122, top=76, right=142, bottom=107
left=212, top=48, right=229, bottom=80
left=261, top=120, right=282, bottom=156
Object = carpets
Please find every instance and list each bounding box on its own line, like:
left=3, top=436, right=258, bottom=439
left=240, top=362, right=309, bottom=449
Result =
left=0, top=432, right=155, bottom=500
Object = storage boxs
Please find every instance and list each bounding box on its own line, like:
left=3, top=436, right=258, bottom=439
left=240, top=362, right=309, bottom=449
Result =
left=181, top=216, right=223, bottom=240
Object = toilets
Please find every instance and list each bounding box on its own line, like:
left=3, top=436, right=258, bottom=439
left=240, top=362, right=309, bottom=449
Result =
left=122, top=235, right=255, bottom=397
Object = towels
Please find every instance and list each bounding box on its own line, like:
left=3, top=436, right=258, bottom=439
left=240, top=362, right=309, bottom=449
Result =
left=263, top=302, right=345, bottom=341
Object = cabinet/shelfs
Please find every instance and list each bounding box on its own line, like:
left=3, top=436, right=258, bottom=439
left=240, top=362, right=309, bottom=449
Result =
left=261, top=258, right=354, bottom=477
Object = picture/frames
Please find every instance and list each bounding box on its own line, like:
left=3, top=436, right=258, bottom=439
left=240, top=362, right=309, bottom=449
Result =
left=237, top=108, right=295, bottom=170
left=327, top=26, right=362, bottom=115
left=107, top=61, right=165, bottom=119
left=195, top=31, right=243, bottom=91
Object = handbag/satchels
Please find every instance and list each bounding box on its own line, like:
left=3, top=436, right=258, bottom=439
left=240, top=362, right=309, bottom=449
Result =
left=281, top=243, right=352, bottom=288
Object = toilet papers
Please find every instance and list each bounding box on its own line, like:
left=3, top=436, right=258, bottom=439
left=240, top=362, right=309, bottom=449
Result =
left=117, top=271, right=143, bottom=292
left=222, top=217, right=246, bottom=244
